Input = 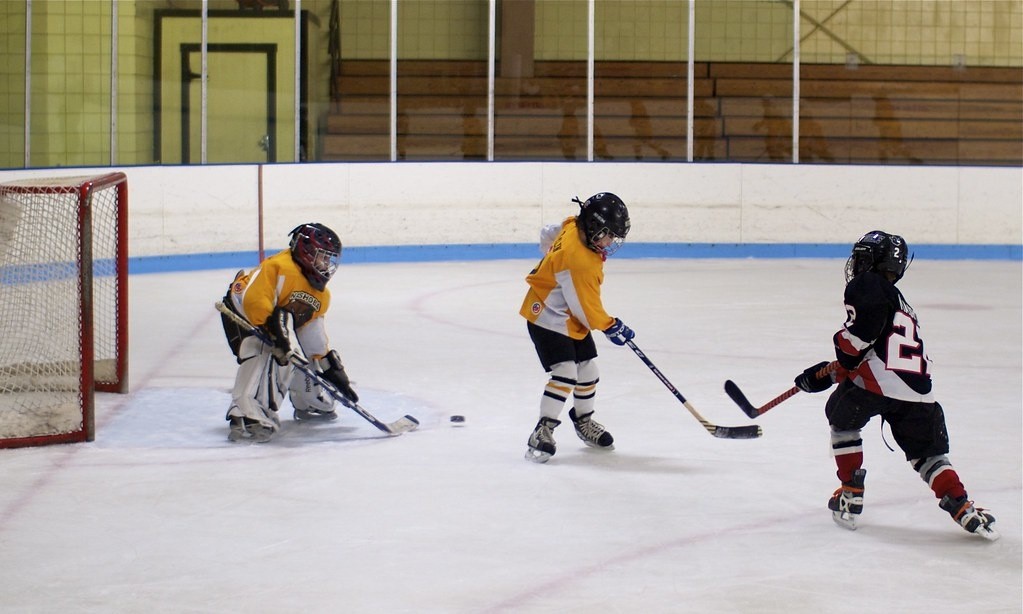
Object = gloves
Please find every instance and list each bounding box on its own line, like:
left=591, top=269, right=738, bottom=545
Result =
left=601, top=318, right=636, bottom=346
left=794, top=362, right=834, bottom=393
left=254, top=316, right=295, bottom=366
left=313, top=349, right=359, bottom=405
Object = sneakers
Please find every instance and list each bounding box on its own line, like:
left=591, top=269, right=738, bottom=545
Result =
left=289, top=394, right=339, bottom=423
left=938, top=492, right=1000, bottom=541
left=525, top=416, right=561, bottom=463
left=568, top=406, right=615, bottom=450
left=829, top=469, right=868, bottom=531
left=227, top=416, right=273, bottom=442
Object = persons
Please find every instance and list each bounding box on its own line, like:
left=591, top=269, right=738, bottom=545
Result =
left=520, top=193, right=636, bottom=462
left=796, top=232, right=998, bottom=541
left=221, top=223, right=358, bottom=443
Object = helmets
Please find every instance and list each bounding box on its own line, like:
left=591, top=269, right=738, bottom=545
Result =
left=571, top=192, right=633, bottom=256
left=288, top=223, right=342, bottom=292
left=845, top=230, right=914, bottom=286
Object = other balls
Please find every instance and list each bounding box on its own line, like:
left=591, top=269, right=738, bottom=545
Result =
left=450, top=415, right=465, bottom=422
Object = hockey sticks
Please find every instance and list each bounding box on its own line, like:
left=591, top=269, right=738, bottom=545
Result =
left=214, top=301, right=420, bottom=438
left=627, top=339, right=763, bottom=441
left=723, top=359, right=841, bottom=420
